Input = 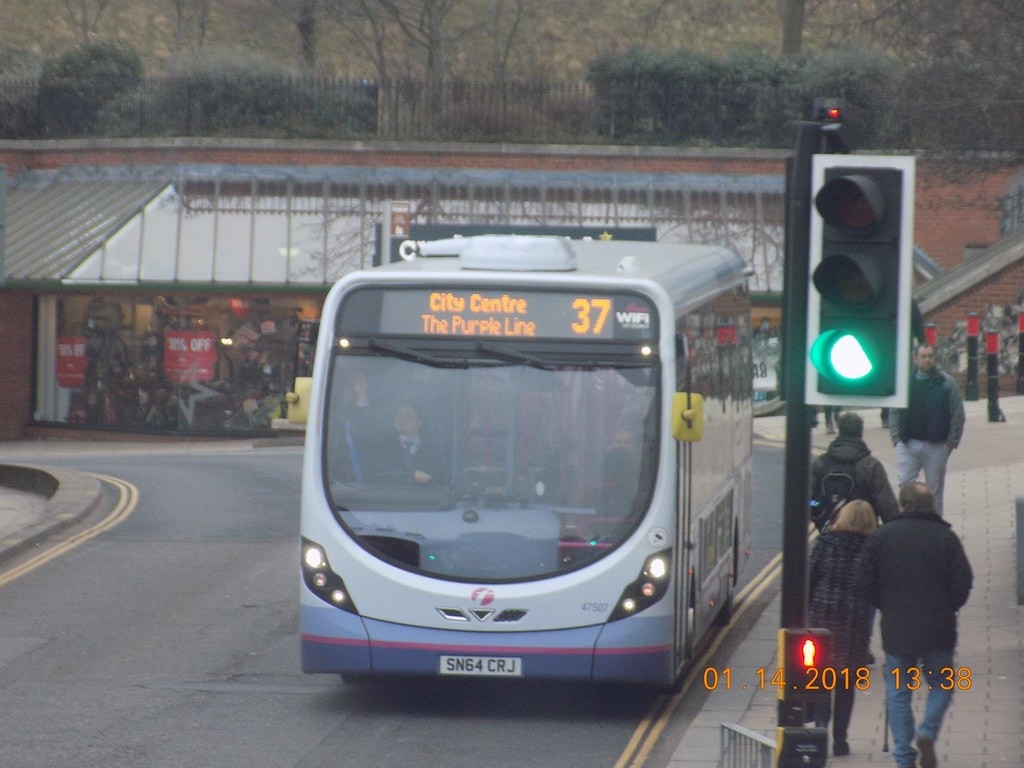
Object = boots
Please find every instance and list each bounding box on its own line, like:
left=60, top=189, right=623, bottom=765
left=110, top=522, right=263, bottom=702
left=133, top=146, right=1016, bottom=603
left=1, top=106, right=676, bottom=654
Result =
left=817, top=702, right=852, bottom=757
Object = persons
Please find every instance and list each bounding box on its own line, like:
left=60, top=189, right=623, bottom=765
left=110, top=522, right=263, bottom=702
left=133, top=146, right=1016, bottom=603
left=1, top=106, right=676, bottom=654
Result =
left=349, top=372, right=444, bottom=500
left=850, top=482, right=973, bottom=767
left=886, top=341, right=965, bottom=520
left=801, top=411, right=901, bottom=529
left=809, top=499, right=879, bottom=756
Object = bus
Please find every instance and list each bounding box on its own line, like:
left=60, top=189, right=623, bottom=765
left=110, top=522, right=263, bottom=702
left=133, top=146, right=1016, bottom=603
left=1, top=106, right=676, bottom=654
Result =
left=284, top=233, right=755, bottom=690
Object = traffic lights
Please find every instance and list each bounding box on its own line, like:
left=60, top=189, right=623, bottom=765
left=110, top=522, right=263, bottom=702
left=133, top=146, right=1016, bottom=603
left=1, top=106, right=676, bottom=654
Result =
left=800, top=151, right=910, bottom=408
left=785, top=628, right=829, bottom=689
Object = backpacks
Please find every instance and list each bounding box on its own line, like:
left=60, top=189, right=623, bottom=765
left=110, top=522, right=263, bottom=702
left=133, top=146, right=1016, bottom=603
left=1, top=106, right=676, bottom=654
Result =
left=822, top=451, right=858, bottom=510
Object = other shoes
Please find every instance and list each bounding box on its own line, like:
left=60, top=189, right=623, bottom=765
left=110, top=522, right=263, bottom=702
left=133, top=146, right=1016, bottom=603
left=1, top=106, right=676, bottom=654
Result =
left=917, top=734, right=938, bottom=767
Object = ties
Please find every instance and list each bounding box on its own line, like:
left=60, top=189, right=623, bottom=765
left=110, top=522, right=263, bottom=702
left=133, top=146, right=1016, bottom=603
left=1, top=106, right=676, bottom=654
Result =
left=402, top=439, right=414, bottom=451
